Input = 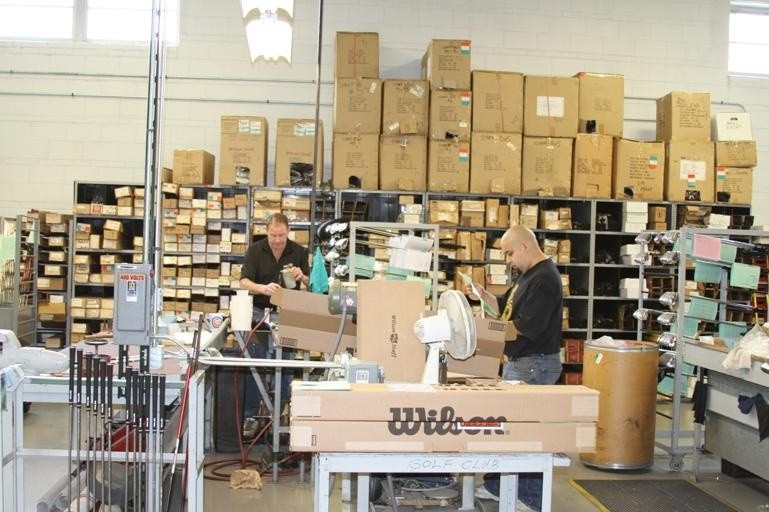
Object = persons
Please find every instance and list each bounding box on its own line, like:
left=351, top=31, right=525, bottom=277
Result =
left=465, top=226, right=563, bottom=512
left=239, top=212, right=309, bottom=437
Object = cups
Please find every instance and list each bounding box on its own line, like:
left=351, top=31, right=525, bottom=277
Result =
left=280, top=268, right=297, bottom=289
left=208, top=312, right=224, bottom=331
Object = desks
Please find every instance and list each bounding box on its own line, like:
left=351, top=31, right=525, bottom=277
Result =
left=311, top=452, right=570, bottom=512
left=17, top=311, right=230, bottom=453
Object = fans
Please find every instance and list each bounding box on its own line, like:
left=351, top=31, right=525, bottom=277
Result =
left=414, top=289, right=476, bottom=381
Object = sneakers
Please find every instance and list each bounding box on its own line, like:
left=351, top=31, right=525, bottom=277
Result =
left=475, top=482, right=499, bottom=502
left=515, top=498, right=538, bottom=511
left=243, top=418, right=259, bottom=439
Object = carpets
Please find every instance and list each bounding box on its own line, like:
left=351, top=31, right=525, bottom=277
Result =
left=568, top=478, right=746, bottom=512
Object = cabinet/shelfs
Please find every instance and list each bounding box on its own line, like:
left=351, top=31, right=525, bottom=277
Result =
left=3, top=183, right=313, bottom=351
left=633, top=231, right=760, bottom=461
left=420, top=205, right=752, bottom=373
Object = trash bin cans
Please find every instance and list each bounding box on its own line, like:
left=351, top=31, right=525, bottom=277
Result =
left=581, top=339, right=660, bottom=470
